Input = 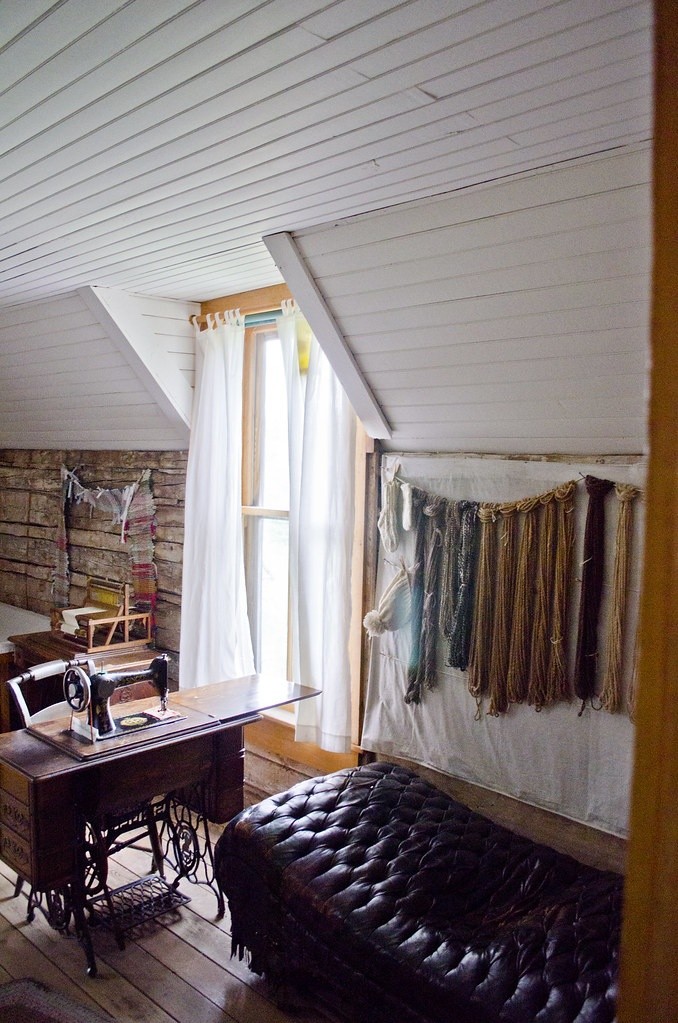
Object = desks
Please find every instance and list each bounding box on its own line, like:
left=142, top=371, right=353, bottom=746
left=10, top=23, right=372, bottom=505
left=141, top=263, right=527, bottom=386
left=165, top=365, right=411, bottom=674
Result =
left=6, top=630, right=172, bottom=729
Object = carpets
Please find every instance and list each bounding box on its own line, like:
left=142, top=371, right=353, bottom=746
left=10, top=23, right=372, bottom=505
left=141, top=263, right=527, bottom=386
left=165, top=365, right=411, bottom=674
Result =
left=0, top=978, right=119, bottom=1023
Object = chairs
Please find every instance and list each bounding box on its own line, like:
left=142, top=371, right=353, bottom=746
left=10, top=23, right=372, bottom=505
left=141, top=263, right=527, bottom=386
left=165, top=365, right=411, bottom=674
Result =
left=5, top=656, right=166, bottom=922
left=47, top=575, right=153, bottom=653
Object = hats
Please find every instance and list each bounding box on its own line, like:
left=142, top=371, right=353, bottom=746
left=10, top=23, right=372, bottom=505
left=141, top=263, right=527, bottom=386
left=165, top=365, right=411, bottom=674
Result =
left=363, top=568, right=413, bottom=637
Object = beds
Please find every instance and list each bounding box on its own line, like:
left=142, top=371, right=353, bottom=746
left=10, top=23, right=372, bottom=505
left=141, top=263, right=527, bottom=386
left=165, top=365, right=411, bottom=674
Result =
left=0, top=601, right=52, bottom=734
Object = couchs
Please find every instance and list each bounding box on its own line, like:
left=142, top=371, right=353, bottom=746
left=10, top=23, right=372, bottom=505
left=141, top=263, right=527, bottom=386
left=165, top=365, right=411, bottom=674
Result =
left=212, top=761, right=626, bottom=1023
